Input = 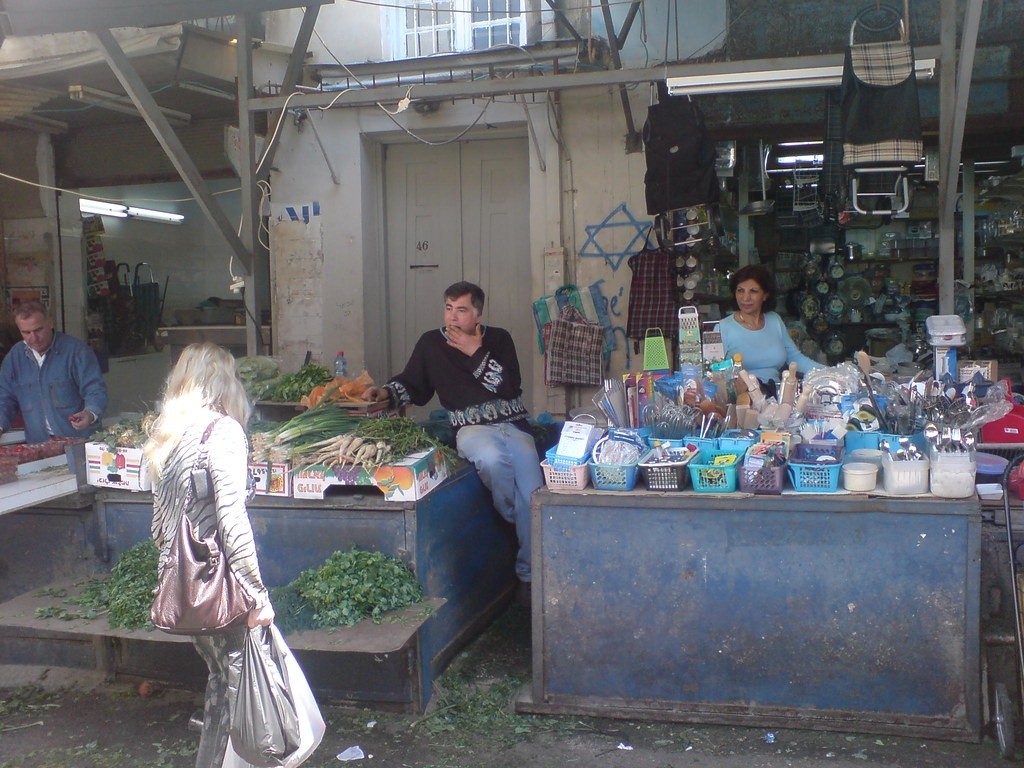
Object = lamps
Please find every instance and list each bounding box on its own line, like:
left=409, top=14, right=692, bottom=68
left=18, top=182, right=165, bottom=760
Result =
left=67, top=84, right=192, bottom=129
left=79, top=197, right=186, bottom=226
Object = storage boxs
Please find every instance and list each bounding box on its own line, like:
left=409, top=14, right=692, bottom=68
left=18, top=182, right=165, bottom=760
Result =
left=82, top=438, right=450, bottom=502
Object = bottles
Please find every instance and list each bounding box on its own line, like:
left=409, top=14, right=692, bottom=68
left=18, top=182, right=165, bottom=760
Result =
left=787, top=249, right=889, bottom=364
left=653, top=439, right=674, bottom=458
left=357, top=359, right=370, bottom=378
left=335, top=351, right=345, bottom=377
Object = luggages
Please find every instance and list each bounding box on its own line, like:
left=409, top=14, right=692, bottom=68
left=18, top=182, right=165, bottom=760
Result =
left=841, top=4, right=924, bottom=217
left=642, top=81, right=725, bottom=247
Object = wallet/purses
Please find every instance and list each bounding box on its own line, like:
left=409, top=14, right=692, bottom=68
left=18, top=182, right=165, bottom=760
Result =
left=191, top=467, right=251, bottom=500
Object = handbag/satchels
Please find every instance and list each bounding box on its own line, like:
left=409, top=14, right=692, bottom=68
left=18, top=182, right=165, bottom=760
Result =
left=532, top=281, right=615, bottom=386
left=150, top=416, right=256, bottom=636
left=220, top=622, right=326, bottom=768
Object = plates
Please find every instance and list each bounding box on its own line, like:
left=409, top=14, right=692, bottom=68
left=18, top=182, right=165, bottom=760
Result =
left=911, top=263, right=938, bottom=301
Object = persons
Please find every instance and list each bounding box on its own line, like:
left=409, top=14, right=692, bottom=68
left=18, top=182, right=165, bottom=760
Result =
left=0, top=301, right=110, bottom=444
left=713, top=264, right=828, bottom=389
left=144, top=342, right=276, bottom=768
left=361, top=281, right=544, bottom=609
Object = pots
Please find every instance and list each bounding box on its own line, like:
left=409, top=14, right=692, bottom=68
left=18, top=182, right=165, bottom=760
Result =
left=838, top=240, right=866, bottom=263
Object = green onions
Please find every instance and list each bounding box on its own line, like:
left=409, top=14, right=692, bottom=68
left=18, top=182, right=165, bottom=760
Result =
left=251, top=383, right=361, bottom=463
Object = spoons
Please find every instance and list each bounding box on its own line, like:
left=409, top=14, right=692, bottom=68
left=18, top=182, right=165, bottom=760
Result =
left=924, top=422, right=938, bottom=453
left=961, top=430, right=975, bottom=454
left=879, top=435, right=924, bottom=462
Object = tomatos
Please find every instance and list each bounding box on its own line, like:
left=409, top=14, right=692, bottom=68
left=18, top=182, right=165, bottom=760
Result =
left=0, top=436, right=85, bottom=484
left=67, top=416, right=77, bottom=421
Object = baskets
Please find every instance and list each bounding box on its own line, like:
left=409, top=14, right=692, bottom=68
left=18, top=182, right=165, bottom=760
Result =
left=541, top=416, right=917, bottom=491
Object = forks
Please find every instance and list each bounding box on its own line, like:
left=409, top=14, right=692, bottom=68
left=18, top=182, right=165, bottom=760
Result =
left=936, top=426, right=962, bottom=453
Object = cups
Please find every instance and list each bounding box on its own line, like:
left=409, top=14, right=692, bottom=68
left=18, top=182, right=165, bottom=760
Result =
left=673, top=203, right=703, bottom=305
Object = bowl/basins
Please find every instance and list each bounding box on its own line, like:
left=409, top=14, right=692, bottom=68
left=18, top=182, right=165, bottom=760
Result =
left=883, top=277, right=900, bottom=287
left=842, top=448, right=884, bottom=491
left=897, top=362, right=926, bottom=376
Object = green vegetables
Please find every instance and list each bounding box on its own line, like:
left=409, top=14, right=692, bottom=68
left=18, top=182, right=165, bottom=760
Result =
left=34, top=538, right=159, bottom=632
left=86, top=415, right=149, bottom=448
left=244, top=364, right=334, bottom=437
left=265, top=546, right=424, bottom=638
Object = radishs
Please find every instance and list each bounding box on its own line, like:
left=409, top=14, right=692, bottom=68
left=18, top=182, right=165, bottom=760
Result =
left=281, top=435, right=391, bottom=473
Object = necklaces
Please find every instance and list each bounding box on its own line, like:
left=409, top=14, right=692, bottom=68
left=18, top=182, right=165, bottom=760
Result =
left=739, top=313, right=752, bottom=328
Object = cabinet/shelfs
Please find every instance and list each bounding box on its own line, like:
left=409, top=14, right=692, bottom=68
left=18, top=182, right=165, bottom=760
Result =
left=803, top=207, right=1003, bottom=366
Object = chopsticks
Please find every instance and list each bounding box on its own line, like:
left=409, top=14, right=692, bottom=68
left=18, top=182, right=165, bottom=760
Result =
left=699, top=413, right=715, bottom=438
